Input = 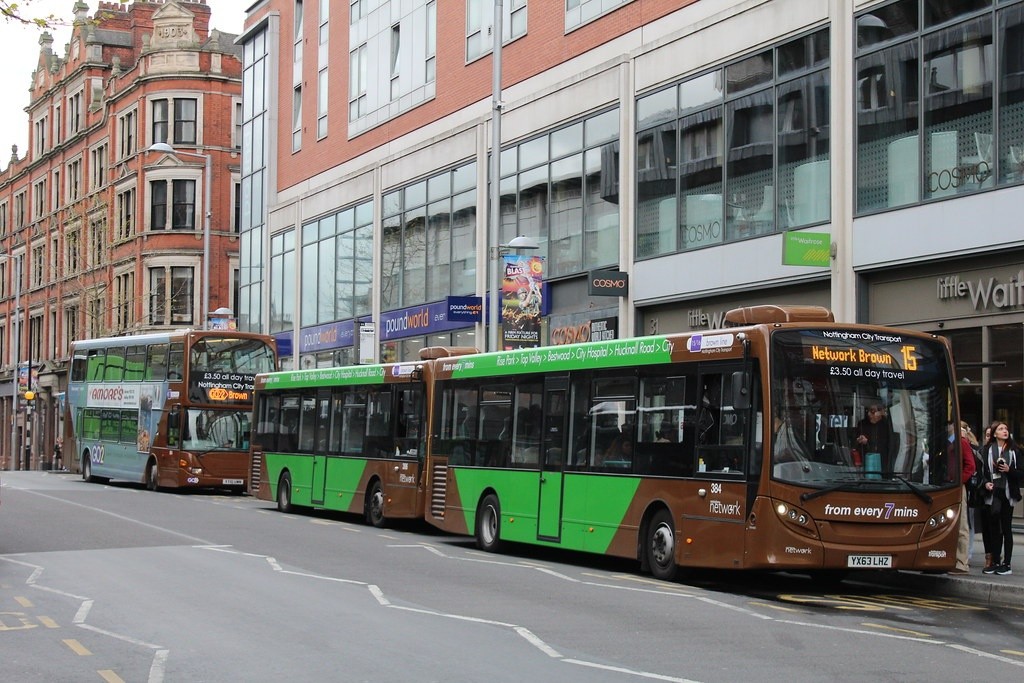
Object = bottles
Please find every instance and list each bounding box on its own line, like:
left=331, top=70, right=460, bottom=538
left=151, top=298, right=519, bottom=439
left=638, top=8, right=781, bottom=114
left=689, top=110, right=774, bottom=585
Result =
left=396, top=447, right=400, bottom=455
left=699, top=458, right=706, bottom=472
left=851, top=449, right=861, bottom=466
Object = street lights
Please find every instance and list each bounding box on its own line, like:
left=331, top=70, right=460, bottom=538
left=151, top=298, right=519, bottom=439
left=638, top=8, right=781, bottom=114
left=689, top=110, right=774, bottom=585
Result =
left=489, top=0, right=543, bottom=354
left=20, top=390, right=35, bottom=471
left=0, top=254, right=40, bottom=470
left=148, top=142, right=235, bottom=368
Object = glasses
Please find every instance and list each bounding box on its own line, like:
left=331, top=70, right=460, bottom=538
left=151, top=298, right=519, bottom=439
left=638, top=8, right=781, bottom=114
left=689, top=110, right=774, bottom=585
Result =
left=865, top=409, right=876, bottom=414
left=865, top=408, right=880, bottom=413
left=946, top=420, right=953, bottom=426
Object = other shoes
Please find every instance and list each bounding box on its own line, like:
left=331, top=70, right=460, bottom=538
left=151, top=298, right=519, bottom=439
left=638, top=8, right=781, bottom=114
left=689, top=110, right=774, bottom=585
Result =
left=982, top=561, right=1001, bottom=574
left=954, top=560, right=970, bottom=571
left=997, top=564, right=1013, bottom=575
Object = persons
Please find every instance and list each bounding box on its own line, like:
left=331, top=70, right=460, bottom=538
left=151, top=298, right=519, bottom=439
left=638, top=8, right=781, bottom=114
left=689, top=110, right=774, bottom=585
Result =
left=55, top=436, right=64, bottom=469
left=853, top=400, right=895, bottom=470
left=933, top=418, right=1005, bottom=573
left=590, top=432, right=634, bottom=467
left=974, top=420, right=1024, bottom=576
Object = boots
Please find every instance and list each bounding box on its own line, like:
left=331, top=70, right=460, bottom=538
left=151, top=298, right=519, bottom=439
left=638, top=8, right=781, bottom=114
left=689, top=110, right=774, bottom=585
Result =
left=983, top=553, right=991, bottom=568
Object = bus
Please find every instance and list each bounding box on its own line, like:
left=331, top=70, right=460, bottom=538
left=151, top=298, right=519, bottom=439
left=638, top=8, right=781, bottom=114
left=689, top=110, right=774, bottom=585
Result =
left=424, top=304, right=964, bottom=583
left=62, top=329, right=280, bottom=494
left=247, top=346, right=482, bottom=529
